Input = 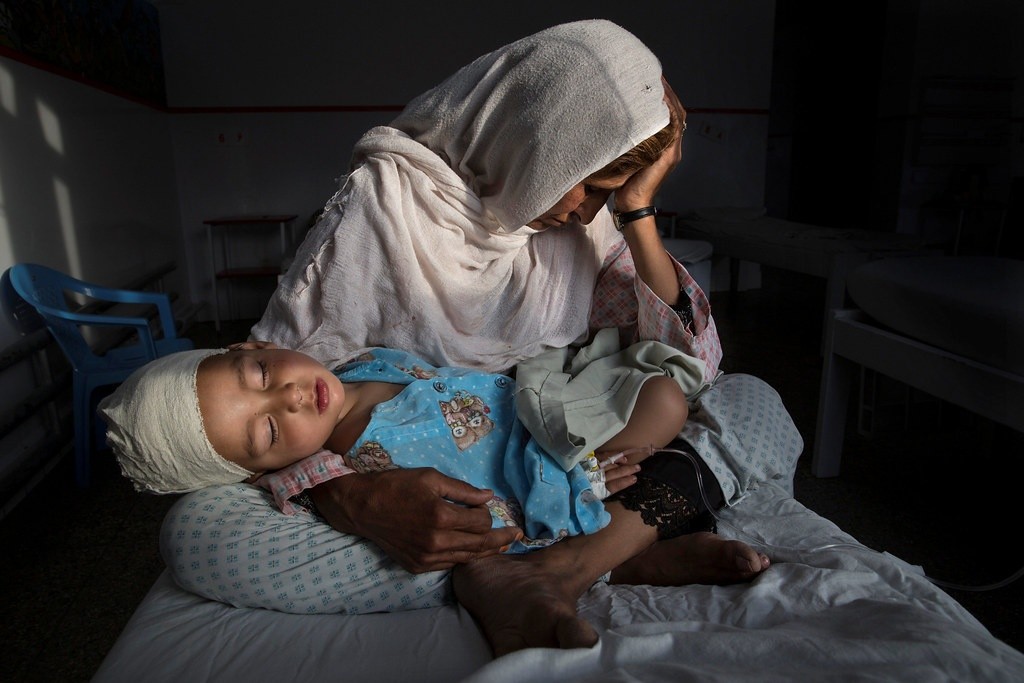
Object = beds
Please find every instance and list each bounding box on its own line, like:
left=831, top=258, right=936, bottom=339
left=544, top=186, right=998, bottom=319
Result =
left=676, top=205, right=944, bottom=357
left=91, top=496, right=1024, bottom=683
left=811, top=242, right=1024, bottom=488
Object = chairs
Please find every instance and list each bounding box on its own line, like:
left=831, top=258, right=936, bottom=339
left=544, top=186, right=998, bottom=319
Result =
left=10, top=262, right=192, bottom=501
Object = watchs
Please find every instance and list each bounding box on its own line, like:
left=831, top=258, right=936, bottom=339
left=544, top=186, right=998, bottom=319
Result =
left=612, top=207, right=658, bottom=230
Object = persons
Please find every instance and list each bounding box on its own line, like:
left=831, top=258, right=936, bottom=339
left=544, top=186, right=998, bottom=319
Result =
left=159, top=18, right=801, bottom=656
left=100, top=340, right=689, bottom=556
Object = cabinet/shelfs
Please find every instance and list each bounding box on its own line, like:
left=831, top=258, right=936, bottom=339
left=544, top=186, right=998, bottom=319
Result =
left=201, top=215, right=300, bottom=331
